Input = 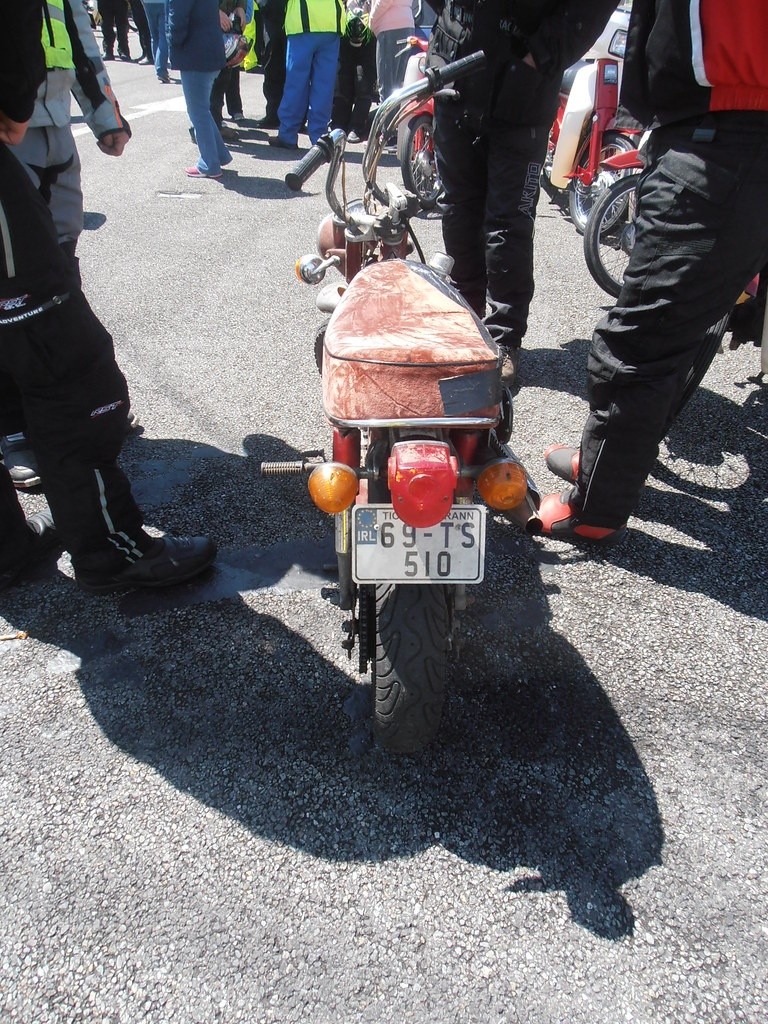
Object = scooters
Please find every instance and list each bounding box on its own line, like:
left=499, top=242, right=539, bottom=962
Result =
left=393, top=28, right=762, bottom=423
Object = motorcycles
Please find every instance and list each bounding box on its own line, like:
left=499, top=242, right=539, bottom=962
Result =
left=257, top=49, right=546, bottom=757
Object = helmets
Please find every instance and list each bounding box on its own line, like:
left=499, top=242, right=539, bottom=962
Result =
left=220, top=12, right=248, bottom=69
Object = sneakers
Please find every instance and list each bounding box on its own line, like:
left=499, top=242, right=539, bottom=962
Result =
left=232, top=111, right=244, bottom=120
left=185, top=166, right=223, bottom=178
left=0, top=508, right=64, bottom=584
left=495, top=343, right=520, bottom=387
left=529, top=493, right=627, bottom=547
left=126, top=412, right=140, bottom=432
left=76, top=535, right=217, bottom=593
left=0, top=431, right=42, bottom=489
left=544, top=444, right=580, bottom=485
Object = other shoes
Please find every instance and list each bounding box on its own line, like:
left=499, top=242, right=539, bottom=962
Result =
left=102, top=52, right=115, bottom=60
left=158, top=75, right=170, bottom=83
left=347, top=129, right=360, bottom=143
left=256, top=117, right=280, bottom=128
left=298, top=119, right=307, bottom=133
left=220, top=127, right=239, bottom=139
left=135, top=55, right=145, bottom=63
left=120, top=52, right=129, bottom=61
left=269, top=136, right=298, bottom=150
left=189, top=126, right=195, bottom=138
left=138, top=57, right=154, bottom=65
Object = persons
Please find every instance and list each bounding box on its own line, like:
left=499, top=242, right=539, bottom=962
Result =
left=364, top=0, right=415, bottom=150
left=503, top=0, right=768, bottom=543
left=268, top=0, right=347, bottom=151
left=129, top=0, right=154, bottom=65
left=4, top=0, right=141, bottom=488
left=200, top=0, right=378, bottom=143
left=423, top=0, right=620, bottom=393
left=0, top=0, right=216, bottom=593
left=140, top=0, right=172, bottom=83
left=97, top=0, right=132, bottom=61
left=166, top=0, right=232, bottom=178
